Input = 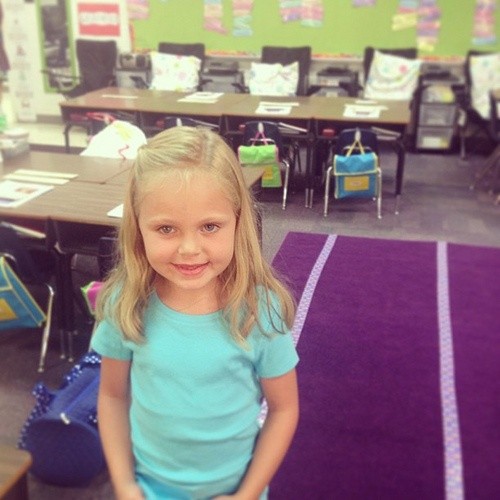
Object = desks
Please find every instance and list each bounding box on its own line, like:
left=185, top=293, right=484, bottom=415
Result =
left=0, top=151, right=264, bottom=227
left=60, top=86, right=415, bottom=215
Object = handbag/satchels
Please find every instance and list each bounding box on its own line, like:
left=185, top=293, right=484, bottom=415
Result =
left=333, top=141, right=378, bottom=201
left=236, top=130, right=283, bottom=189
left=15, top=351, right=110, bottom=488
left=1, top=257, right=50, bottom=329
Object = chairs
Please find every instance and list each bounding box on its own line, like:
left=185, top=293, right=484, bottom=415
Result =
left=64, top=40, right=500, bottom=159
left=0, top=222, right=55, bottom=373
left=242, top=119, right=291, bottom=210
left=323, top=130, right=384, bottom=218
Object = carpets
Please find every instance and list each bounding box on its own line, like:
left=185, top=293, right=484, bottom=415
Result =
left=269, top=231, right=500, bottom=500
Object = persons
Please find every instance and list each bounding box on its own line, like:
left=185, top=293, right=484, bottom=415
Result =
left=90, top=126, right=301, bottom=500
